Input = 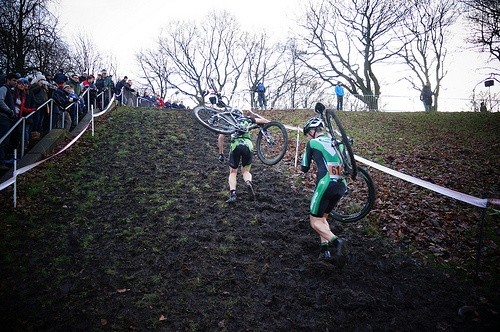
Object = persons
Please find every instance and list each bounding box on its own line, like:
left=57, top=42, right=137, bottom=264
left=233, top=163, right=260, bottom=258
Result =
left=300, top=116, right=349, bottom=264
left=218, top=110, right=270, bottom=203
left=255, top=82, right=267, bottom=110
left=420, top=83, right=433, bottom=111
left=334, top=81, right=345, bottom=111
left=0, top=69, right=190, bottom=175
left=479, top=102, right=487, bottom=112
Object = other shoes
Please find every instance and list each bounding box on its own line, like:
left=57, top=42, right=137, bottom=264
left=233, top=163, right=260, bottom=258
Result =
left=0, top=164, right=10, bottom=170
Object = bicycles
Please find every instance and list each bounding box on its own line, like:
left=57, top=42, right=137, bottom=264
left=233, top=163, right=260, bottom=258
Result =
left=325, top=108, right=376, bottom=224
left=192, top=89, right=288, bottom=166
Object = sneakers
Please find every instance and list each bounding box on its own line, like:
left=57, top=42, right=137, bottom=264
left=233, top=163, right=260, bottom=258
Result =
left=332, top=238, right=343, bottom=255
left=226, top=197, right=236, bottom=203
left=245, top=181, right=253, bottom=188
left=319, top=251, right=331, bottom=258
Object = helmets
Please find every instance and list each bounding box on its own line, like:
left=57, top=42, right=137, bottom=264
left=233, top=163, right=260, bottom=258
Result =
left=232, top=108, right=244, bottom=113
left=303, top=116, right=324, bottom=135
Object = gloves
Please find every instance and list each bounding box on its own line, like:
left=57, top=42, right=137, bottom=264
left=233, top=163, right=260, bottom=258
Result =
left=218, top=153, right=224, bottom=164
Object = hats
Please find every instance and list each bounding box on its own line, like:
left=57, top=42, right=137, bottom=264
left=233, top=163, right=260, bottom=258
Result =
left=36, top=72, right=46, bottom=82
left=62, top=85, right=69, bottom=89
